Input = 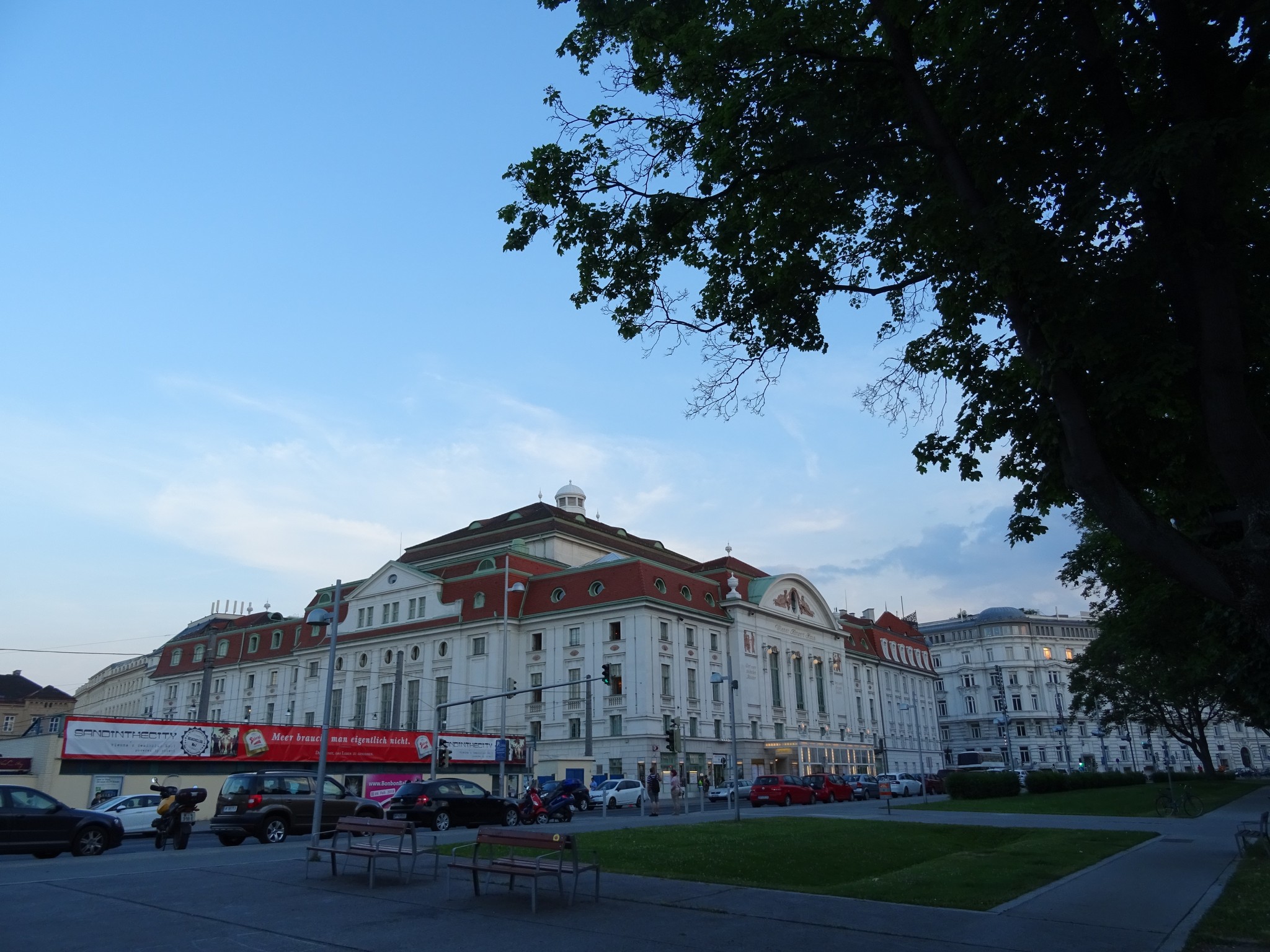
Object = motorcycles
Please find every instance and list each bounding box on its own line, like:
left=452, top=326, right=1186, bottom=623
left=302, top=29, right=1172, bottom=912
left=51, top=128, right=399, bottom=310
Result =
left=150, top=774, right=207, bottom=851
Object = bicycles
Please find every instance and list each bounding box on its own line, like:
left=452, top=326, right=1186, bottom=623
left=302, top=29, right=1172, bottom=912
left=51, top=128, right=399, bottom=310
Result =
left=1155, top=784, right=1203, bottom=817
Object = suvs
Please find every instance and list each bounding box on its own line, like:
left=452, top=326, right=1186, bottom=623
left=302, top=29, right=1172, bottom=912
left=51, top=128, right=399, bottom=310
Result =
left=538, top=779, right=589, bottom=811
left=210, top=769, right=384, bottom=844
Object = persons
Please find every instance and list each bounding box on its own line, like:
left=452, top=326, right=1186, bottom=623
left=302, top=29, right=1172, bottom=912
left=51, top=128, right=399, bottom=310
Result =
left=647, top=767, right=662, bottom=816
left=698, top=775, right=710, bottom=796
left=744, top=630, right=755, bottom=653
left=511, top=741, right=526, bottom=758
left=670, top=769, right=680, bottom=816
left=1218, top=765, right=1225, bottom=772
left=406, top=778, right=432, bottom=783
left=211, top=731, right=239, bottom=755
left=719, top=776, right=725, bottom=784
left=102, top=793, right=112, bottom=796
left=590, top=777, right=597, bottom=791
left=1197, top=764, right=1203, bottom=773
left=833, top=653, right=841, bottom=672
left=773, top=587, right=815, bottom=618
left=91, top=792, right=105, bottom=806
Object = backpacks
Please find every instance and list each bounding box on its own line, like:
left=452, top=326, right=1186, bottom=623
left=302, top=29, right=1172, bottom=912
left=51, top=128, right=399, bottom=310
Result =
left=705, top=779, right=710, bottom=785
left=648, top=773, right=660, bottom=793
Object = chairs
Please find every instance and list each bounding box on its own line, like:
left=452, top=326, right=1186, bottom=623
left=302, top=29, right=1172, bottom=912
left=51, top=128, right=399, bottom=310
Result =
left=285, top=782, right=291, bottom=792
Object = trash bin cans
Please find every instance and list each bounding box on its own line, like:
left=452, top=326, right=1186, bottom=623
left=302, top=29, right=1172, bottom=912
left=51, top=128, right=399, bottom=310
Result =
left=878, top=779, right=892, bottom=799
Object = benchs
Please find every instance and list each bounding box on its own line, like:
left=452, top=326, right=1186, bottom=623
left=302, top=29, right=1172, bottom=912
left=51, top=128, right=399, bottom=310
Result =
left=447, top=825, right=601, bottom=916
left=305, top=816, right=439, bottom=892
left=1234, top=811, right=1269, bottom=859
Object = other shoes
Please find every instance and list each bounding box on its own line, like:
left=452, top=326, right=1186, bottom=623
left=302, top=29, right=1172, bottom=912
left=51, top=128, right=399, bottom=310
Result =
left=649, top=812, right=658, bottom=816
left=671, top=813, right=679, bottom=815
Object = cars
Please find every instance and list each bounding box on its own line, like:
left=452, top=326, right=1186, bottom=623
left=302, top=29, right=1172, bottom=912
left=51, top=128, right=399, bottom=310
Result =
left=589, top=778, right=646, bottom=810
left=708, top=773, right=942, bottom=807
left=386, top=778, right=520, bottom=831
left=0, top=784, right=124, bottom=859
left=937, top=769, right=1083, bottom=786
left=86, top=794, right=161, bottom=835
left=1234, top=767, right=1270, bottom=778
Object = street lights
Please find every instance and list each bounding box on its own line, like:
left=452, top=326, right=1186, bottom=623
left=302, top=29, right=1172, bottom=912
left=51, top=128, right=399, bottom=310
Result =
left=306, top=579, right=341, bottom=861
left=900, top=691, right=927, bottom=804
left=710, top=656, right=740, bottom=822
left=499, top=555, right=525, bottom=798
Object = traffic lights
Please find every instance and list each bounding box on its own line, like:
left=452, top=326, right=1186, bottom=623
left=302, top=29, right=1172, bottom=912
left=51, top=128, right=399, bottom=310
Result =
left=1059, top=741, right=1063, bottom=747
left=601, top=662, right=612, bottom=686
left=1053, top=764, right=1055, bottom=768
left=439, top=739, right=446, bottom=746
left=507, top=678, right=516, bottom=699
left=1079, top=758, right=1082, bottom=766
left=1216, top=753, right=1221, bottom=758
left=443, top=749, right=452, bottom=768
left=1187, top=753, right=1191, bottom=761
left=1155, top=752, right=1160, bottom=761
left=670, top=716, right=680, bottom=725
left=438, top=749, right=446, bottom=768
left=665, top=729, right=676, bottom=752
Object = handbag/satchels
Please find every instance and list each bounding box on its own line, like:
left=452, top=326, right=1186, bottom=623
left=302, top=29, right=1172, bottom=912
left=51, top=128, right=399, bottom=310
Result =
left=679, top=788, right=683, bottom=796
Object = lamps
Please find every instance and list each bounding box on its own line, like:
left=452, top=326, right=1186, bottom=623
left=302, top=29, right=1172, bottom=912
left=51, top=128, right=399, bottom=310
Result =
left=147, top=713, right=153, bottom=720
left=243, top=715, right=249, bottom=723
left=810, top=657, right=818, bottom=681
left=399, top=725, right=404, bottom=729
left=788, top=653, right=796, bottom=677
left=763, top=648, right=772, bottom=673
left=372, top=712, right=378, bottom=721
left=287, top=708, right=293, bottom=716
left=830, top=661, right=838, bottom=685
left=189, top=702, right=199, bottom=712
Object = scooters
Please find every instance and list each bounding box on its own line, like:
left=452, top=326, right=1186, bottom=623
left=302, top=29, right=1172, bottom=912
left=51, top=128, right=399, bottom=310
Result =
left=518, top=778, right=575, bottom=825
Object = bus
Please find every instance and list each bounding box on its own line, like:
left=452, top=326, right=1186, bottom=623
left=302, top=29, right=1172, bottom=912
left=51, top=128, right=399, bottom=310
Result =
left=957, top=751, right=1004, bottom=771
